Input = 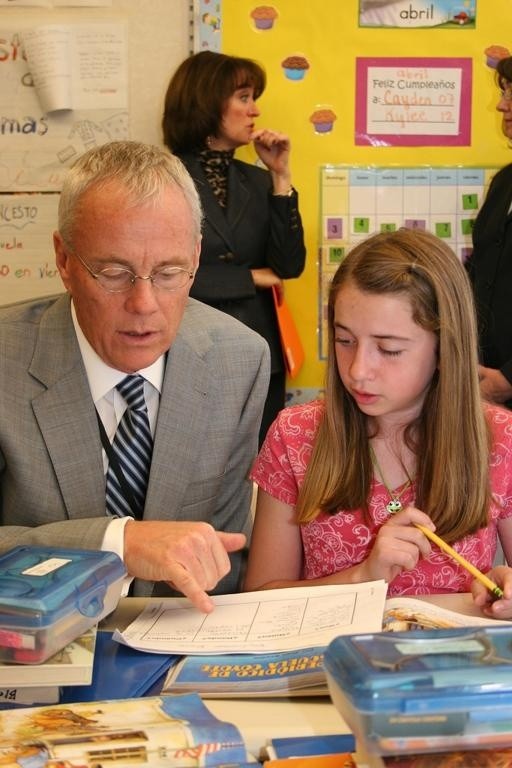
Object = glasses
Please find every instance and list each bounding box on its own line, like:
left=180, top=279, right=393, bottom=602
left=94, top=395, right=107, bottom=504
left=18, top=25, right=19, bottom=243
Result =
left=60, top=239, right=198, bottom=293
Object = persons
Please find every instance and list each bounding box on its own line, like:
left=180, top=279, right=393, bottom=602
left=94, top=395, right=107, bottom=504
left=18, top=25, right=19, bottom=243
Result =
left=0, top=140, right=271, bottom=611
left=242, top=228, right=512, bottom=619
left=161, top=50, right=306, bottom=454
left=465, top=56, right=512, bottom=410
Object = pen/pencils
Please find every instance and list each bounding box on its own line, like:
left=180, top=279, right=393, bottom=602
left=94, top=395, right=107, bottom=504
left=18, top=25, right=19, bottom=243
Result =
left=415, top=525, right=504, bottom=599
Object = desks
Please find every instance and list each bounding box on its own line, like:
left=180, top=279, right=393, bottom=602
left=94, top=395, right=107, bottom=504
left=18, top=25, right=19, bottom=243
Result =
left=1, top=594, right=511, bottom=768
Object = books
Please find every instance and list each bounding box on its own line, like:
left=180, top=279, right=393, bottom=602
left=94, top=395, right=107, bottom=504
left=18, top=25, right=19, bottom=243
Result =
left=159, top=597, right=512, bottom=701
left=0, top=623, right=98, bottom=690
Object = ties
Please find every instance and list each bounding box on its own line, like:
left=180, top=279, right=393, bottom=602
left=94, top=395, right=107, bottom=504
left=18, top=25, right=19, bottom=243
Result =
left=104, top=373, right=154, bottom=521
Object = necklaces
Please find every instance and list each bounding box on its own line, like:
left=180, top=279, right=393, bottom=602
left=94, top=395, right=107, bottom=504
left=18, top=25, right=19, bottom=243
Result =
left=369, top=440, right=418, bottom=515
